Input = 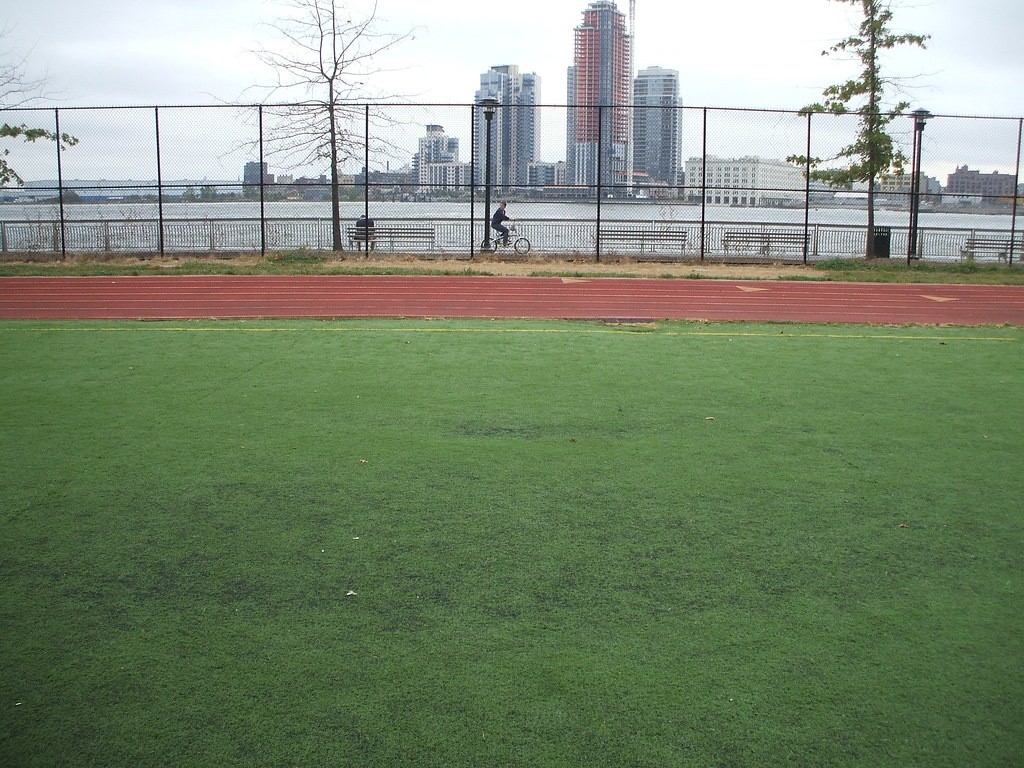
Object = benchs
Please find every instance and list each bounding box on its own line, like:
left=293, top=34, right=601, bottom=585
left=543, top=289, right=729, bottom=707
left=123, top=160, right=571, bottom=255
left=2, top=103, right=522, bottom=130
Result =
left=347, top=228, right=436, bottom=254
left=960, top=238, right=1024, bottom=262
left=722, top=232, right=810, bottom=260
left=595, top=229, right=687, bottom=256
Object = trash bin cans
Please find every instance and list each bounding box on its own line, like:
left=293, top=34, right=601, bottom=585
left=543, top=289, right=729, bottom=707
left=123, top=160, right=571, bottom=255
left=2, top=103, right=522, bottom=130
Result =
left=873, top=226, right=891, bottom=258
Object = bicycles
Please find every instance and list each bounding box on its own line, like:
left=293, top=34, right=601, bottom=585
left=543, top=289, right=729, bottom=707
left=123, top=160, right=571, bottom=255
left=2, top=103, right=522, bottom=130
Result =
left=481, top=219, right=531, bottom=255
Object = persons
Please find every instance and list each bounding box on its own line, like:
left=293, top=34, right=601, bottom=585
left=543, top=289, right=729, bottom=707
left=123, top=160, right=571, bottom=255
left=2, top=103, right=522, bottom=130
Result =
left=354, top=215, right=377, bottom=252
left=491, top=202, right=513, bottom=246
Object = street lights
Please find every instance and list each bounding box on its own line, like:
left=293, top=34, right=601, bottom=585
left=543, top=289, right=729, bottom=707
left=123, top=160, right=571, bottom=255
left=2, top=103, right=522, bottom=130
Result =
left=908, top=107, right=934, bottom=260
left=475, top=97, right=503, bottom=250
